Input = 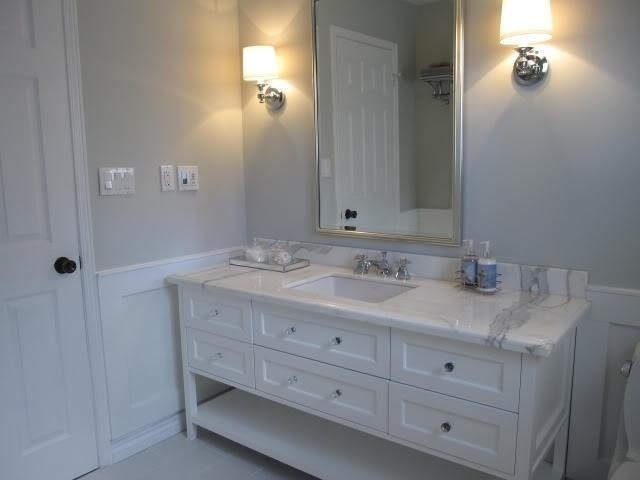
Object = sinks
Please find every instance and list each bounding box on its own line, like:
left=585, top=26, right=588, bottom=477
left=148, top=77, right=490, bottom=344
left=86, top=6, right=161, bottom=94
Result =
left=283, top=273, right=420, bottom=309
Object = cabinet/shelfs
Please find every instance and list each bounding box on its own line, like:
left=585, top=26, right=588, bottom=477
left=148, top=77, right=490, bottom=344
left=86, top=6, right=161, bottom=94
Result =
left=188, top=328, right=255, bottom=389
left=197, top=384, right=557, bottom=479
left=252, top=298, right=391, bottom=378
left=253, top=345, right=390, bottom=436
left=391, top=329, right=526, bottom=415
left=180, top=287, right=252, bottom=339
left=387, top=382, right=520, bottom=479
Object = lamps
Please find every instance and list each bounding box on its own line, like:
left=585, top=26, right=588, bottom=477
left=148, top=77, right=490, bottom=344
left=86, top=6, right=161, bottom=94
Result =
left=497, top=0, right=555, bottom=87
left=241, top=44, right=286, bottom=112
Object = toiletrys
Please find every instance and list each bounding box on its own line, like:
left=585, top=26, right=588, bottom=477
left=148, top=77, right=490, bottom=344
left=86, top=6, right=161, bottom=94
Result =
left=476, top=241, right=498, bottom=293
left=460, top=237, right=477, bottom=285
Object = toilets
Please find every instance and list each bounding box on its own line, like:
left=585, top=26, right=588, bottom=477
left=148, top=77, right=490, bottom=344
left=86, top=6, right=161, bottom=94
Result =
left=605, top=339, right=639, bottom=479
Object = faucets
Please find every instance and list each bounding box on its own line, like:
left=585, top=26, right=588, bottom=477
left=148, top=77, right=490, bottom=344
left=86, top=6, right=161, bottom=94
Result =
left=363, top=251, right=393, bottom=277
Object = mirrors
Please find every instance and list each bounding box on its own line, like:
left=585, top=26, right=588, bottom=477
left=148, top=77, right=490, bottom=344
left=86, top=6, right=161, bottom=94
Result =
left=310, top=0, right=466, bottom=249
